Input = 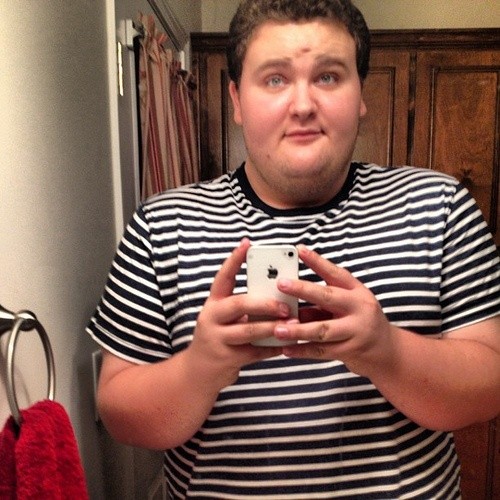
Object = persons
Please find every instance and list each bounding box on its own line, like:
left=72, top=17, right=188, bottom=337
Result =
left=83, top=0, right=500, bottom=500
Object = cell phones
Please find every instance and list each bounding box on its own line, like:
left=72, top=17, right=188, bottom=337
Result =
left=247, top=245, right=300, bottom=347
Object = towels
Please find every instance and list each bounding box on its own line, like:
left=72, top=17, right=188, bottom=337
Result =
left=0, top=399, right=90, bottom=500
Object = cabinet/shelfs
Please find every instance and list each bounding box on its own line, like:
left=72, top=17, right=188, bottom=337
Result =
left=190, top=28, right=500, bottom=500
